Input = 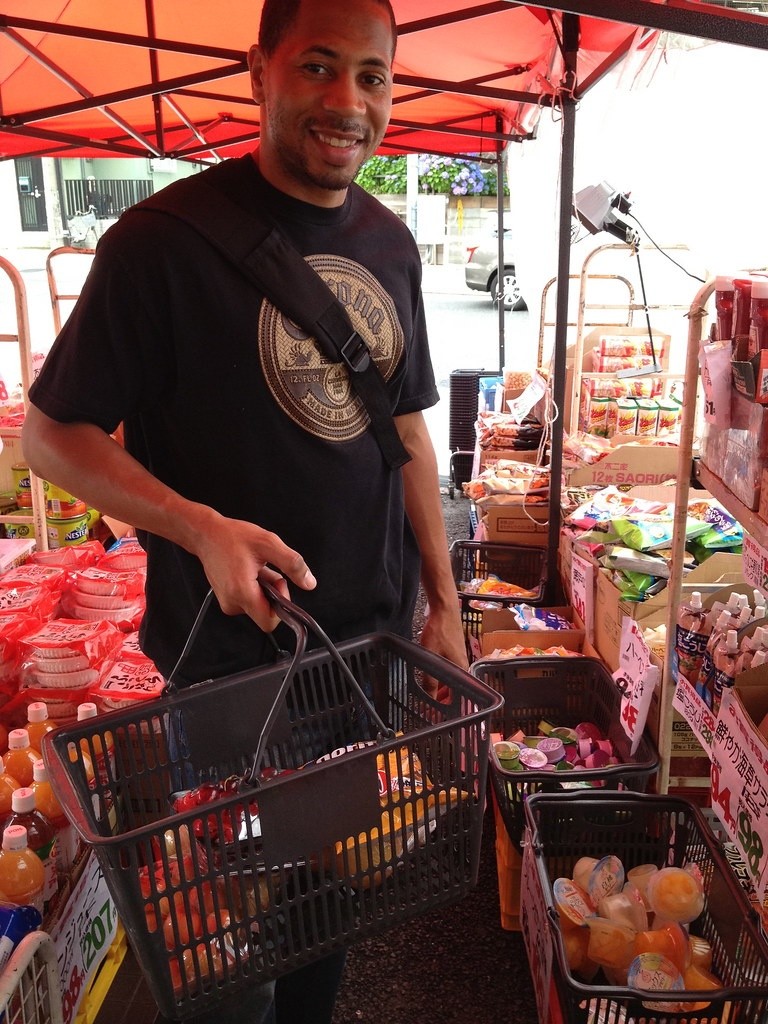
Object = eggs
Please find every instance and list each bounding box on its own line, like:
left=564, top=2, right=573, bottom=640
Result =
left=135, top=857, right=253, bottom=982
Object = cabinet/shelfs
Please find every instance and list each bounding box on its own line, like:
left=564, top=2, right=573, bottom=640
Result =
left=657, top=276, right=768, bottom=1023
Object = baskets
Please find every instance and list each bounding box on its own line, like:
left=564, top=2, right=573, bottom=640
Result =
left=39, top=577, right=499, bottom=1022
left=447, top=537, right=768, bottom=1024
left=449, top=368, right=500, bottom=490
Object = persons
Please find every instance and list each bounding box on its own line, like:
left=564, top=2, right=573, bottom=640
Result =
left=20, top=0, right=469, bottom=1024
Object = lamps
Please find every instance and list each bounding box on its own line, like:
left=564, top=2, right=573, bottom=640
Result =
left=571, top=181, right=641, bottom=250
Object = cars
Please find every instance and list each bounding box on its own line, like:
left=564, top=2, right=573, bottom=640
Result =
left=465, top=228, right=527, bottom=313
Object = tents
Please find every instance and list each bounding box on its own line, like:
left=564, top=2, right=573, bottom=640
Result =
left=0, top=1, right=768, bottom=606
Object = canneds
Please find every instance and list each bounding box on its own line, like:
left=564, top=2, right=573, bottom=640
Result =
left=0, top=462, right=101, bottom=550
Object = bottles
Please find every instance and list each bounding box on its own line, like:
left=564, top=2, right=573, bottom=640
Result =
left=0, top=698, right=117, bottom=934
left=714, top=271, right=768, bottom=361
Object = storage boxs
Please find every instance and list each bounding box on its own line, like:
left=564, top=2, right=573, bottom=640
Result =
left=470, top=377, right=550, bottom=546
left=563, top=325, right=681, bottom=487
left=490, top=778, right=622, bottom=930
left=31, top=728, right=172, bottom=935
left=698, top=349, right=768, bottom=522
left=0, top=539, right=37, bottom=574
left=558, top=485, right=768, bottom=1024
left=0, top=427, right=25, bottom=492
left=482, top=605, right=585, bottom=719
